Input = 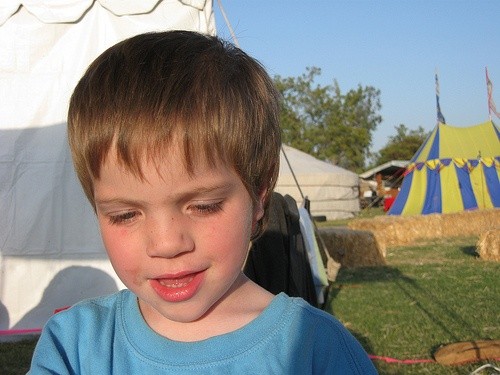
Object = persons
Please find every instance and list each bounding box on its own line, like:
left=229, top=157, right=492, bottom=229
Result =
left=25, top=29, right=380, bottom=374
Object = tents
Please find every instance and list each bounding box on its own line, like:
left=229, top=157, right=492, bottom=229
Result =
left=385, top=119, right=500, bottom=216
left=357, top=158, right=408, bottom=205
left=0, top=0, right=223, bottom=342
left=272, top=142, right=362, bottom=224
